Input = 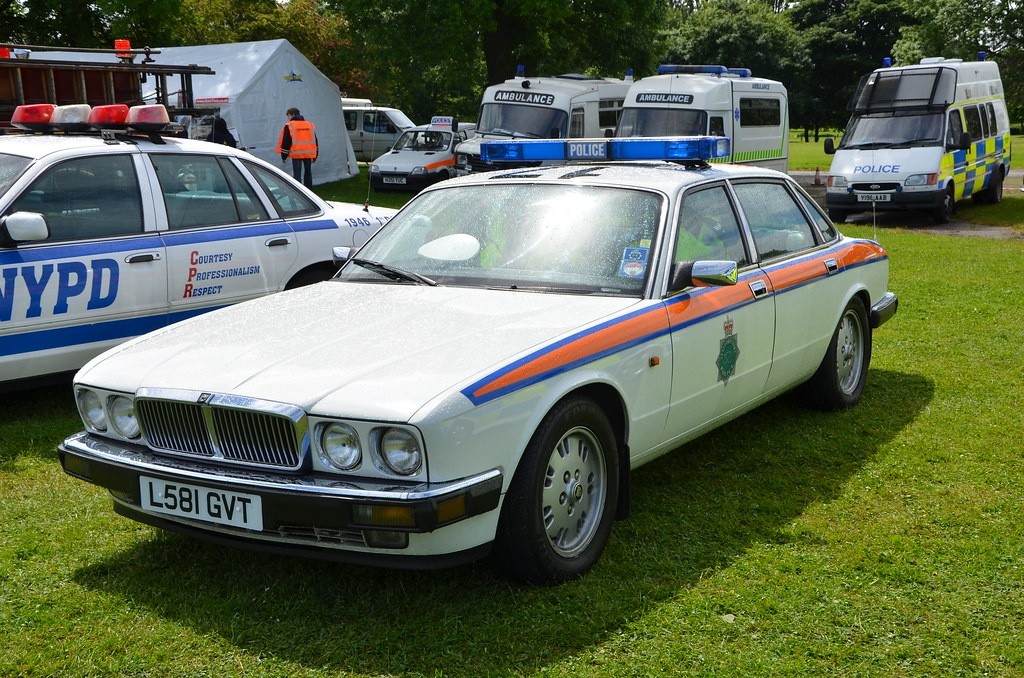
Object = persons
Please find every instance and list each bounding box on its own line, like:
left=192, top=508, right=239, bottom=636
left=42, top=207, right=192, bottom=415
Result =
left=276, top=108, right=319, bottom=191
left=207, top=118, right=237, bottom=148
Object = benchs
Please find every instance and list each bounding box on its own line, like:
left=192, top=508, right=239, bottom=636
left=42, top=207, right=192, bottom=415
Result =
left=710, top=228, right=806, bottom=262
left=165, top=192, right=255, bottom=227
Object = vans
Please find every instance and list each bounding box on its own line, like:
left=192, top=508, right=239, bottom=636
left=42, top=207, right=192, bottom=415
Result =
left=824, top=51, right=1012, bottom=225
left=339, top=98, right=435, bottom=162
left=450, top=65, right=640, bottom=177
left=603, top=65, right=789, bottom=179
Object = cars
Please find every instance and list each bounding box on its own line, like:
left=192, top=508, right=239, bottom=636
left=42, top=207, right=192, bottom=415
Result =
left=57, top=130, right=899, bottom=589
left=0, top=104, right=432, bottom=386
left=368, top=116, right=477, bottom=195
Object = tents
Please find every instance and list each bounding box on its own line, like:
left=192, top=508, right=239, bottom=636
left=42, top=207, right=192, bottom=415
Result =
left=10, top=39, right=360, bottom=186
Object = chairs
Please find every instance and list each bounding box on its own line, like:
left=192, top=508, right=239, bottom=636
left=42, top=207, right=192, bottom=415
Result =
left=41, top=170, right=76, bottom=213
left=92, top=177, right=129, bottom=215
